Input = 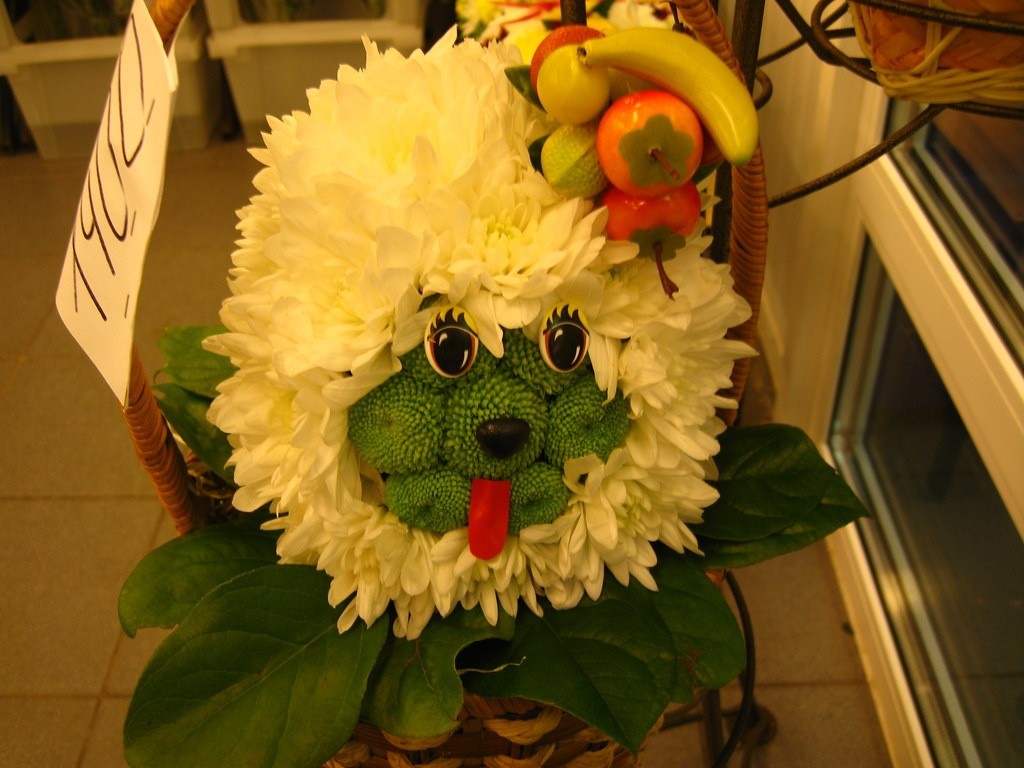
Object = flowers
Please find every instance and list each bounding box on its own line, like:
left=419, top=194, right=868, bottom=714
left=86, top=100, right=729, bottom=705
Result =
left=116, top=24, right=875, bottom=768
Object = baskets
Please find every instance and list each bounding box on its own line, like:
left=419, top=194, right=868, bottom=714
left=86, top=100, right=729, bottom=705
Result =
left=122, top=0, right=765, bottom=722
left=848, top=0, right=1024, bottom=104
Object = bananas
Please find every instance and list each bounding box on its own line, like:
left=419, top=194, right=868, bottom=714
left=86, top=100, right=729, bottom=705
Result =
left=575, top=28, right=758, bottom=166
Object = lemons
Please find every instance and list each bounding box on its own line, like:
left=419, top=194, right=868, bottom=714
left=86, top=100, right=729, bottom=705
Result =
left=536, top=44, right=659, bottom=126
